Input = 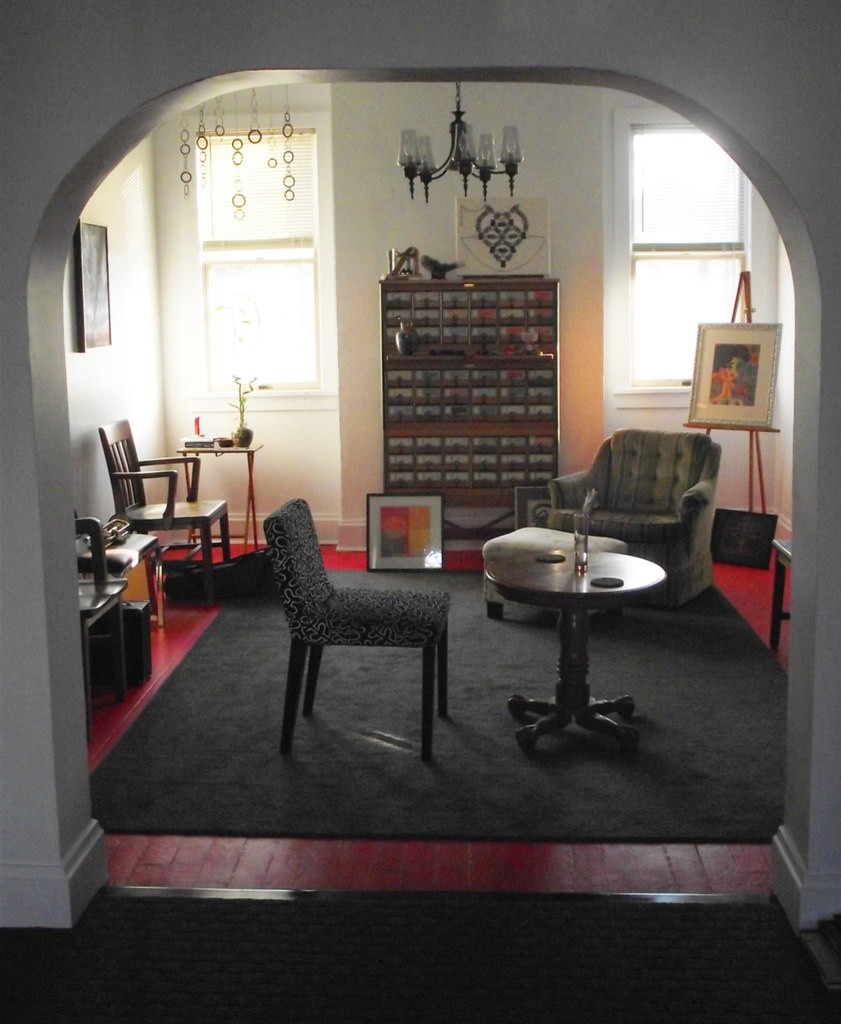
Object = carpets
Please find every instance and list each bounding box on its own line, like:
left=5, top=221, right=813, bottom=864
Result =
left=90, top=569, right=788, bottom=843
left=0, top=891, right=841, bottom=1024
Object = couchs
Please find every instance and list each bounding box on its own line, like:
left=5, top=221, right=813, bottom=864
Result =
left=547, top=429, right=721, bottom=608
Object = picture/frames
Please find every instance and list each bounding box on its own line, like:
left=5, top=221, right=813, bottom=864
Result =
left=79, top=218, right=111, bottom=352
left=710, top=507, right=779, bottom=570
left=366, top=492, right=444, bottom=572
left=688, top=323, right=783, bottom=426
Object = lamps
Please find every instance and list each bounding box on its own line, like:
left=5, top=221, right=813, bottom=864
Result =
left=398, top=80, right=522, bottom=203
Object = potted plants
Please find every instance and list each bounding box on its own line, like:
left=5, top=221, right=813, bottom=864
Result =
left=228, top=375, right=257, bottom=447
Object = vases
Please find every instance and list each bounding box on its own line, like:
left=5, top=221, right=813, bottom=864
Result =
left=395, top=316, right=419, bottom=357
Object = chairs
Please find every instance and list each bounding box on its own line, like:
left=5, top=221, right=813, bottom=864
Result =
left=263, top=498, right=451, bottom=763
left=98, top=419, right=230, bottom=566
left=75, top=517, right=128, bottom=742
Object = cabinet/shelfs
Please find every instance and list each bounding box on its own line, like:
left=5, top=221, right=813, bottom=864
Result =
left=380, top=278, right=558, bottom=506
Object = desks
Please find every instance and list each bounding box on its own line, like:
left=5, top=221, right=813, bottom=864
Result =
left=176, top=443, right=263, bottom=554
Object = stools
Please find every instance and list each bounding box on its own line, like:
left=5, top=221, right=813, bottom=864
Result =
left=770, top=538, right=792, bottom=649
left=77, top=532, right=164, bottom=627
left=482, top=527, right=628, bottom=620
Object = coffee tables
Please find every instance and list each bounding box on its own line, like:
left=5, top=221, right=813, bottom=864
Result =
left=484, top=552, right=667, bottom=765
